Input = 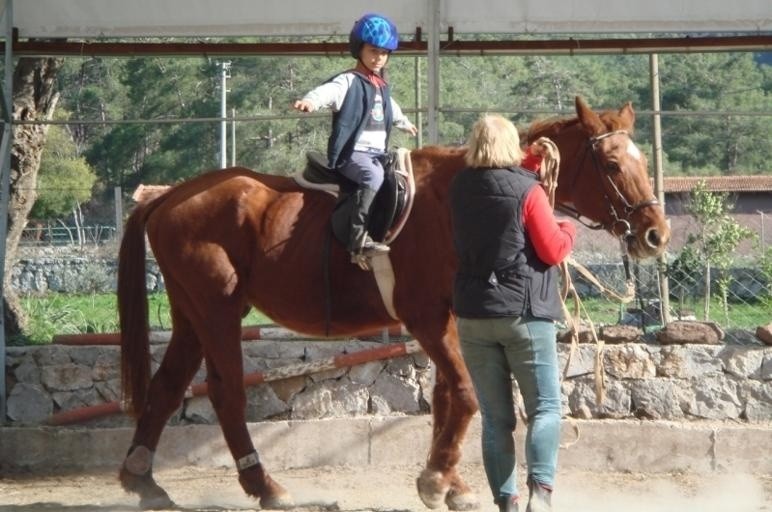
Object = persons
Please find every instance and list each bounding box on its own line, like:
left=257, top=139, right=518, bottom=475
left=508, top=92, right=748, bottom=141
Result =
left=292, top=12, right=420, bottom=271
left=447, top=112, right=580, bottom=512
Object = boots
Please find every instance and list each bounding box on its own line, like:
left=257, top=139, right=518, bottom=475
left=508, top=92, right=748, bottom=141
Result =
left=497, top=494, right=520, bottom=511
left=346, top=186, right=390, bottom=255
left=525, top=477, right=551, bottom=511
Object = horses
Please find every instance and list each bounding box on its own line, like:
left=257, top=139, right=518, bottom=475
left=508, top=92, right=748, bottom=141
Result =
left=111, top=94, right=674, bottom=510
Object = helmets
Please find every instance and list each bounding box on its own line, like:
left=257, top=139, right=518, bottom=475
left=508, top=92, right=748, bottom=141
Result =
left=351, top=14, right=400, bottom=58
